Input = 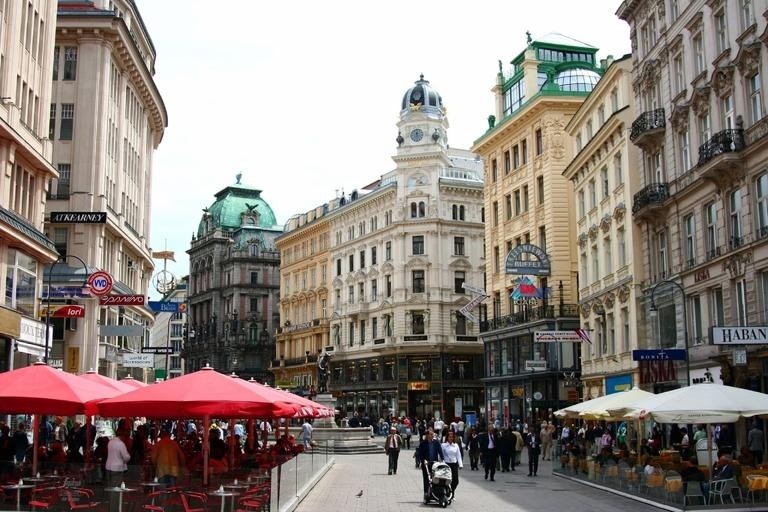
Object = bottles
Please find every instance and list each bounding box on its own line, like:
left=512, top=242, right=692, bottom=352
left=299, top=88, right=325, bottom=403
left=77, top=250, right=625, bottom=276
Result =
left=219, top=469, right=268, bottom=491
left=121, top=476, right=158, bottom=490
left=18, top=469, right=57, bottom=485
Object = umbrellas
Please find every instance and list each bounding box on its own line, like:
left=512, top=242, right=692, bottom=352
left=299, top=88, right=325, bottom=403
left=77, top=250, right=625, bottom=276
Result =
left=605, top=370, right=768, bottom=481
left=552, top=384, right=657, bottom=448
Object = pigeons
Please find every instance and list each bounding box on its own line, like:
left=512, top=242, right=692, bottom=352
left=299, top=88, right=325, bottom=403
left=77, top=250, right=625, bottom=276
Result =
left=355, top=490, right=363, bottom=498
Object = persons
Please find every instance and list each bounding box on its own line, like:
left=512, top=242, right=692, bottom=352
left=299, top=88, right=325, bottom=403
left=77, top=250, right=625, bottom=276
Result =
left=378, top=413, right=765, bottom=504
left=1, top=417, right=314, bottom=512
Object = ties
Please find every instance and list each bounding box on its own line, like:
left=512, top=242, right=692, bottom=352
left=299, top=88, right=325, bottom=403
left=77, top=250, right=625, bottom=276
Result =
left=393, top=436, right=396, bottom=448
left=490, top=433, right=496, bottom=446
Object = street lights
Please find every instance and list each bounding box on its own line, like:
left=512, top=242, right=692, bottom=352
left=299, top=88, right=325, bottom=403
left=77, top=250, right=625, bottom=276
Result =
left=163, top=310, right=197, bottom=382
left=648, top=277, right=692, bottom=386
left=42, top=253, right=92, bottom=367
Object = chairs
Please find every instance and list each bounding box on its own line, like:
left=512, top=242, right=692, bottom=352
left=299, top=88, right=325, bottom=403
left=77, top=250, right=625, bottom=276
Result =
left=1, top=431, right=306, bottom=511
left=554, top=445, right=767, bottom=510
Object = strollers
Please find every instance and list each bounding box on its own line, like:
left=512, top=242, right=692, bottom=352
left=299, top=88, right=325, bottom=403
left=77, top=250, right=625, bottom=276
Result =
left=423, top=461, right=454, bottom=508
